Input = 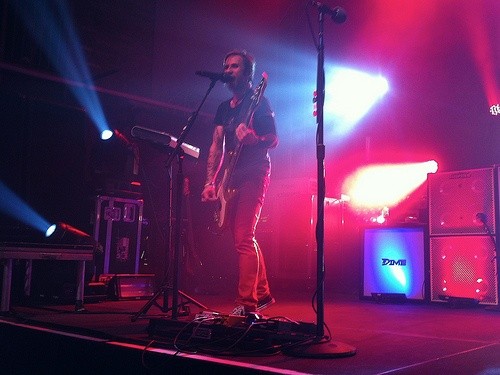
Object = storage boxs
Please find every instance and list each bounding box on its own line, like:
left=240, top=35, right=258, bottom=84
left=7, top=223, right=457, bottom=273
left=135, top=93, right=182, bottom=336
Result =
left=89, top=195, right=143, bottom=274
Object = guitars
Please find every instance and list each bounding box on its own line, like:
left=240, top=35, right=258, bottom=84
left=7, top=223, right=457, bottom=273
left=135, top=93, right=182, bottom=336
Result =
left=208, top=72, right=267, bottom=236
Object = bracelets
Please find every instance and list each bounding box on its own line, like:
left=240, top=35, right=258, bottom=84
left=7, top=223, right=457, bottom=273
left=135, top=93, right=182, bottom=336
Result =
left=203, top=182, right=215, bottom=186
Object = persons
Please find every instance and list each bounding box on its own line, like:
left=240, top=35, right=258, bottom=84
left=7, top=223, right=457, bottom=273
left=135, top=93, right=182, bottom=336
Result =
left=201, top=51, right=279, bottom=315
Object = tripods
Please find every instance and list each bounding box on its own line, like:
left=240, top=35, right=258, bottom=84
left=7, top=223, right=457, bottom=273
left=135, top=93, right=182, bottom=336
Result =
left=129, top=153, right=209, bottom=323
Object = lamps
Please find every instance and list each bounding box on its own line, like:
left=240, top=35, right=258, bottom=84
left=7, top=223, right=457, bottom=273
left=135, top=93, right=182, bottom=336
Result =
left=107, top=275, right=159, bottom=301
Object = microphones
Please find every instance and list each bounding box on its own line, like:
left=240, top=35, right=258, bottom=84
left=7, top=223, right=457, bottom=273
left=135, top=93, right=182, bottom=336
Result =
left=476, top=212, right=486, bottom=222
left=195, top=70, right=232, bottom=83
left=309, top=0, right=347, bottom=22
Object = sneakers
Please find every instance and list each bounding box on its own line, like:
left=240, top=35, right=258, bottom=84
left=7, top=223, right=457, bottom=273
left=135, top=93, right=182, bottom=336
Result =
left=256, top=295, right=275, bottom=311
left=232, top=305, right=248, bottom=315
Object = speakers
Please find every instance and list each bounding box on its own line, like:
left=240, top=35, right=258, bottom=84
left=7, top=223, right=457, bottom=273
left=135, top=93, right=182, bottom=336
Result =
left=362, top=165, right=500, bottom=311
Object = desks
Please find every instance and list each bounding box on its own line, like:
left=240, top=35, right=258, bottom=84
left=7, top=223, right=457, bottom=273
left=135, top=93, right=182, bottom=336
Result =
left=0, top=246, right=92, bottom=311
